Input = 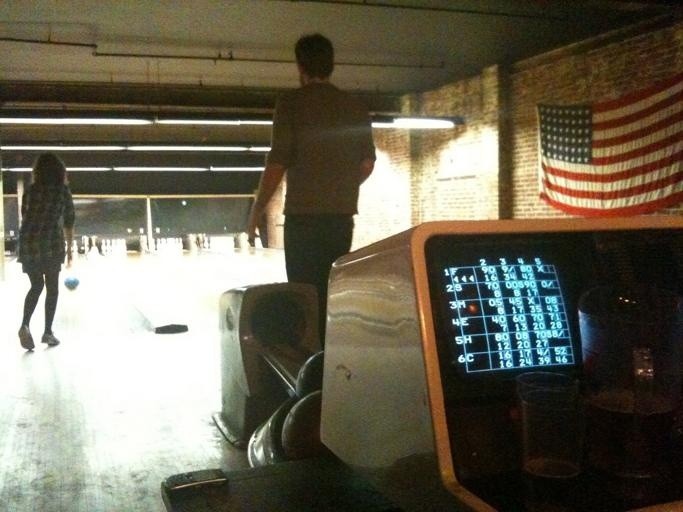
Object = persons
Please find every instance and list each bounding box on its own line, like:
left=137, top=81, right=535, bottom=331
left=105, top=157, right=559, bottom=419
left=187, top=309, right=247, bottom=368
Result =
left=15, top=153, right=75, bottom=351
left=248, top=32, right=376, bottom=349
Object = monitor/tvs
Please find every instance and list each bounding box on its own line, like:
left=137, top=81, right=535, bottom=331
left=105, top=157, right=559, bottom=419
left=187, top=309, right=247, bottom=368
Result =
left=439, top=250, right=584, bottom=395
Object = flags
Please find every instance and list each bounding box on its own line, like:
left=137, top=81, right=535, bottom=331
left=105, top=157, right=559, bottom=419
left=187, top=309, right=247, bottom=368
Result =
left=536, top=72, right=683, bottom=217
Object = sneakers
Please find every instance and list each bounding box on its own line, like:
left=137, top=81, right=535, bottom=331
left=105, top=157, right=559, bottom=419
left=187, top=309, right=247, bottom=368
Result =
left=41, top=332, right=60, bottom=346
left=18, top=325, right=35, bottom=350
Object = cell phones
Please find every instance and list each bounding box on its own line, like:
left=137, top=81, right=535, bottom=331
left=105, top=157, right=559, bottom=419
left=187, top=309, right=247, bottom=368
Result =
left=162, top=468, right=230, bottom=495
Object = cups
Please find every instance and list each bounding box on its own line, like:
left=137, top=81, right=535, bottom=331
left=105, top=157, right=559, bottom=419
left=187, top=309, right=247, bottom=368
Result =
left=518, top=288, right=682, bottom=479
